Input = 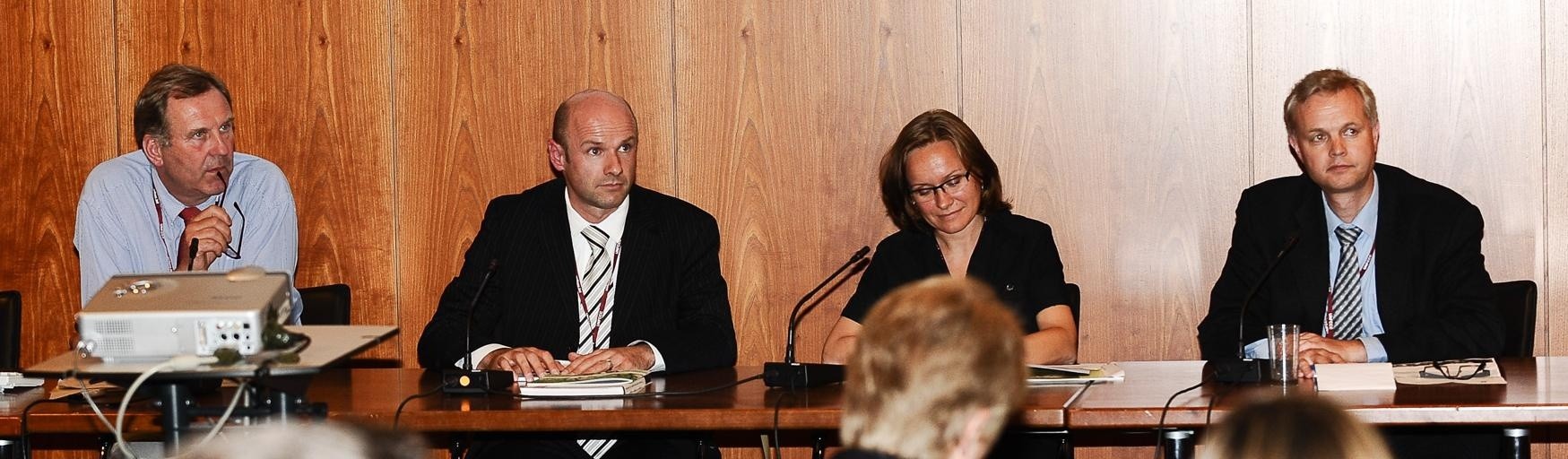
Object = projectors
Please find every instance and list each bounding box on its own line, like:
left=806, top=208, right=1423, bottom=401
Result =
left=71, top=266, right=298, bottom=365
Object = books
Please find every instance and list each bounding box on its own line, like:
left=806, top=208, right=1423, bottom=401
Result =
left=1024, top=363, right=1125, bottom=383
left=517, top=370, right=651, bottom=395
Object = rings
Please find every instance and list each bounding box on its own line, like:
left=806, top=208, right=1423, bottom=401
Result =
left=606, top=359, right=614, bottom=369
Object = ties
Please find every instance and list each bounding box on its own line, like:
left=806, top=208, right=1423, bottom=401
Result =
left=572, top=225, right=619, bottom=459
left=178, top=207, right=201, bottom=228
left=1332, top=226, right=1363, bottom=339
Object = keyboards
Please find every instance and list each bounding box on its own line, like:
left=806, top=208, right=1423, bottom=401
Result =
left=0, top=370, right=46, bottom=391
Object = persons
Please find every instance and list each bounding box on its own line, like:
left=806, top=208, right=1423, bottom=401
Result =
left=823, top=109, right=1079, bottom=365
left=417, top=89, right=737, bottom=459
left=1198, top=389, right=1396, bottom=459
left=72, top=62, right=304, bottom=325
left=1196, top=68, right=1503, bottom=378
left=827, top=274, right=1026, bottom=458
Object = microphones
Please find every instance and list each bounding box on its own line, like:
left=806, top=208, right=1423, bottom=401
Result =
left=1209, top=231, right=1299, bottom=388
left=439, top=254, right=515, bottom=396
left=762, top=245, right=872, bottom=392
left=185, top=234, right=204, bottom=270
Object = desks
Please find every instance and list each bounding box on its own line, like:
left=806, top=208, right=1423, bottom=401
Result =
left=0, top=367, right=1088, bottom=458
left=1067, top=355, right=1567, bottom=458
left=22, top=325, right=408, bottom=433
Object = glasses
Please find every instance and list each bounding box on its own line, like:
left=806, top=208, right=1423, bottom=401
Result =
left=907, top=166, right=971, bottom=205
left=1419, top=360, right=1493, bottom=380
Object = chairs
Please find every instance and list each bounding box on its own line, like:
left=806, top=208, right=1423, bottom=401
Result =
left=1492, top=281, right=1537, bottom=357
left=0, top=290, right=21, bottom=373
left=296, top=284, right=351, bottom=326
left=1066, top=282, right=1080, bottom=363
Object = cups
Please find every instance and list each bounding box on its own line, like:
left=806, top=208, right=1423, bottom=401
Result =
left=1264, top=323, right=1301, bottom=385
